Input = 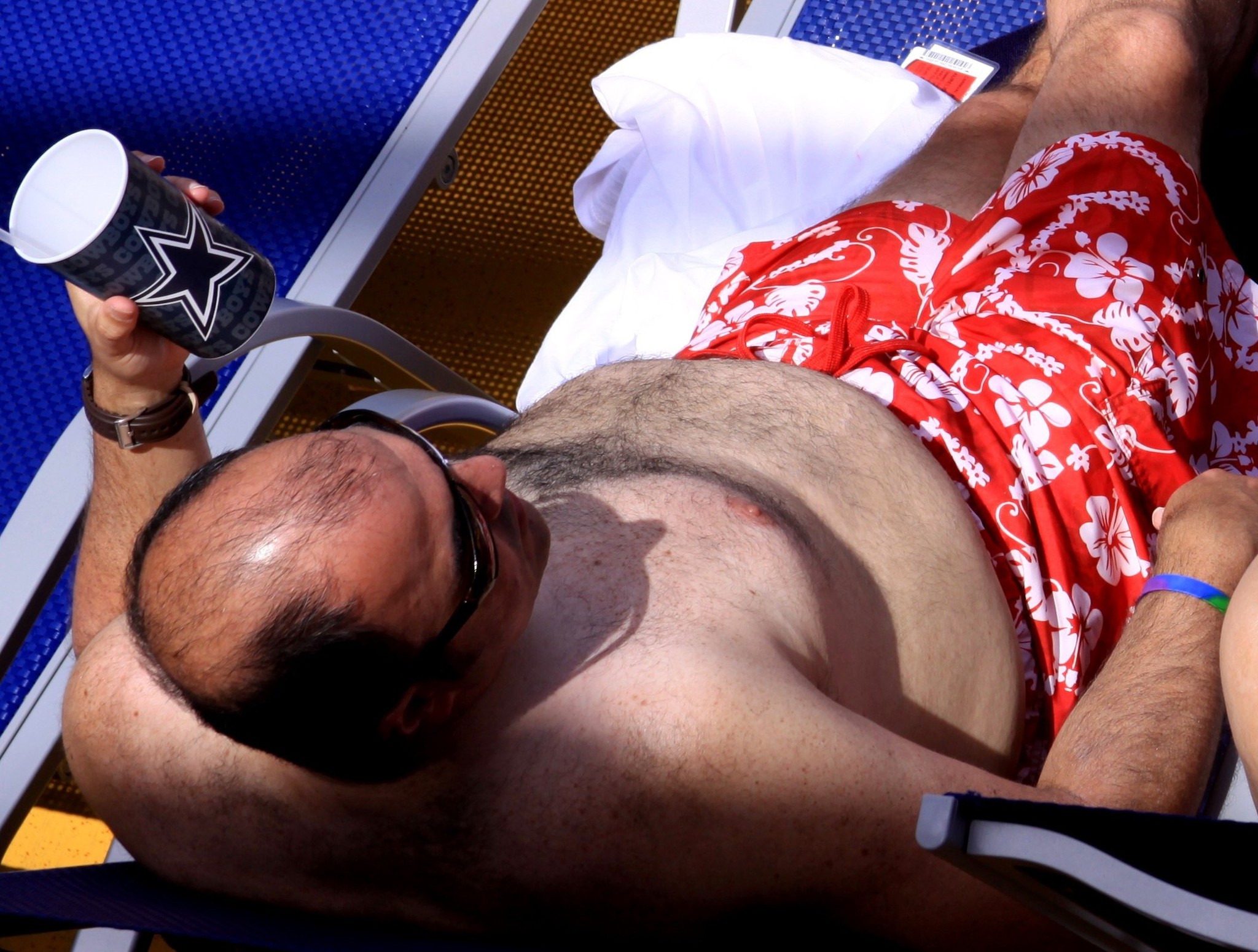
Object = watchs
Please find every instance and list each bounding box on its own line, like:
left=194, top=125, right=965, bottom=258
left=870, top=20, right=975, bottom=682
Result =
left=80, top=358, right=198, bottom=450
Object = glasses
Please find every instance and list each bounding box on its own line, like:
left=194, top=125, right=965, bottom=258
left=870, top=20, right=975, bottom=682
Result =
left=316, top=407, right=498, bottom=718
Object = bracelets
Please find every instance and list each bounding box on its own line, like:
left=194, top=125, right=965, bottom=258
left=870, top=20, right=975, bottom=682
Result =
left=1134, top=573, right=1231, bottom=614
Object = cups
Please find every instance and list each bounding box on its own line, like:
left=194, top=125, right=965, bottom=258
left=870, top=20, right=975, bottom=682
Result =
left=8, top=127, right=277, bottom=361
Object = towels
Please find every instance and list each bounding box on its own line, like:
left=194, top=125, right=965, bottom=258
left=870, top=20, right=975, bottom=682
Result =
left=569, top=33, right=959, bottom=355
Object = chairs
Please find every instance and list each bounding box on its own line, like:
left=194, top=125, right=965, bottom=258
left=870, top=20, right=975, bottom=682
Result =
left=0, top=0, right=1258, bottom=951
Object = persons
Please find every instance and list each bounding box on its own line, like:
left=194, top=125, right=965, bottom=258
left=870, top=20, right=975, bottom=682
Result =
left=57, top=0, right=1258, bottom=952
left=1217, top=555, right=1258, bottom=810
